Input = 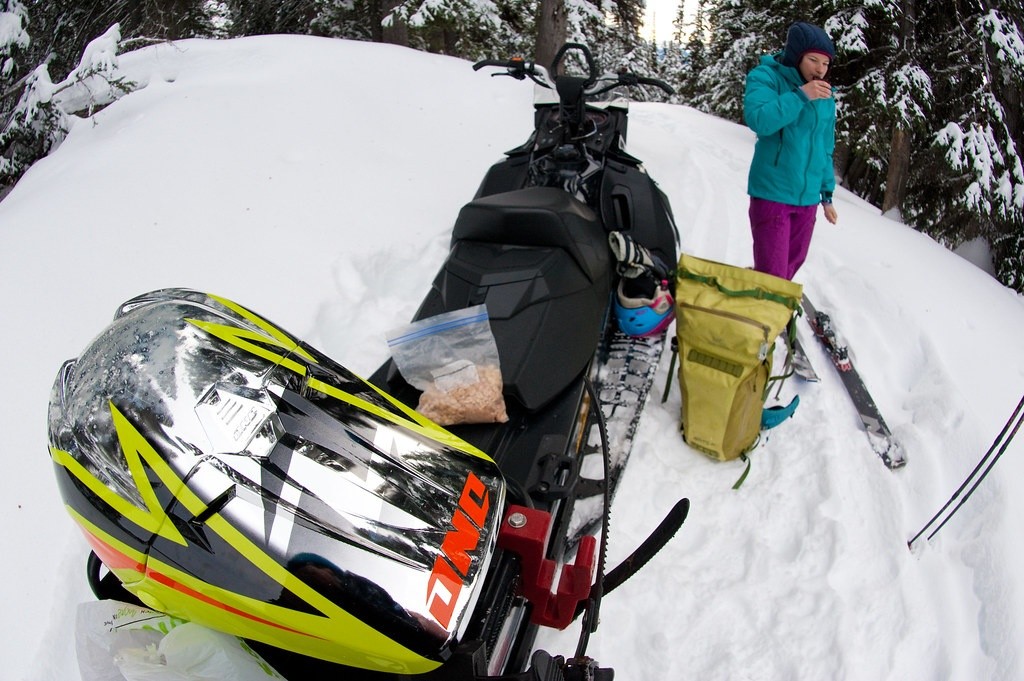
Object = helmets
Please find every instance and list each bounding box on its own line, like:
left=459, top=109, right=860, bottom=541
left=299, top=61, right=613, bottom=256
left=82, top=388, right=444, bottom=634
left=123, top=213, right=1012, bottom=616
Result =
left=614, top=289, right=675, bottom=340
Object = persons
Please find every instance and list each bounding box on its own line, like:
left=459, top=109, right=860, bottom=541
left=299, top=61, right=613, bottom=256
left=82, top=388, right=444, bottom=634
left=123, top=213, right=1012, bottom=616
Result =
left=742, top=24, right=838, bottom=279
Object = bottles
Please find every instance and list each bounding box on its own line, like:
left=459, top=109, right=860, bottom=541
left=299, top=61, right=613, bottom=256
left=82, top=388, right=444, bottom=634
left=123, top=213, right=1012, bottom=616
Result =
left=653, top=280, right=669, bottom=299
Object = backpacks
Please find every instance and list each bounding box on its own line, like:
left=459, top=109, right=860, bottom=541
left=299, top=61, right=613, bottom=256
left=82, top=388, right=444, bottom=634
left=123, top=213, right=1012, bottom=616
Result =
left=674, top=255, right=803, bottom=461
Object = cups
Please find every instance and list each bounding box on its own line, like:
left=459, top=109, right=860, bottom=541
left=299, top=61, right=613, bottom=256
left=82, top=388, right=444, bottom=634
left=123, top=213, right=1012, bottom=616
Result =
left=814, top=76, right=831, bottom=100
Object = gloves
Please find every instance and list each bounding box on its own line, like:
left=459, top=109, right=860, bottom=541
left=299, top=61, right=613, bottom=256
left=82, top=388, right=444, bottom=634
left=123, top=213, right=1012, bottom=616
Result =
left=609, top=231, right=670, bottom=284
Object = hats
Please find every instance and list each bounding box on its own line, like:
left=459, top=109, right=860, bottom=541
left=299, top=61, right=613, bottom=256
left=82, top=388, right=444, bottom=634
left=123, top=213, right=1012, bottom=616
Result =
left=782, top=22, right=836, bottom=67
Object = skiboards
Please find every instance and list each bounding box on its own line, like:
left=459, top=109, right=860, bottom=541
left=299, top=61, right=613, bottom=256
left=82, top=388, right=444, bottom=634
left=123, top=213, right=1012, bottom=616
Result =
left=744, top=264, right=909, bottom=471
